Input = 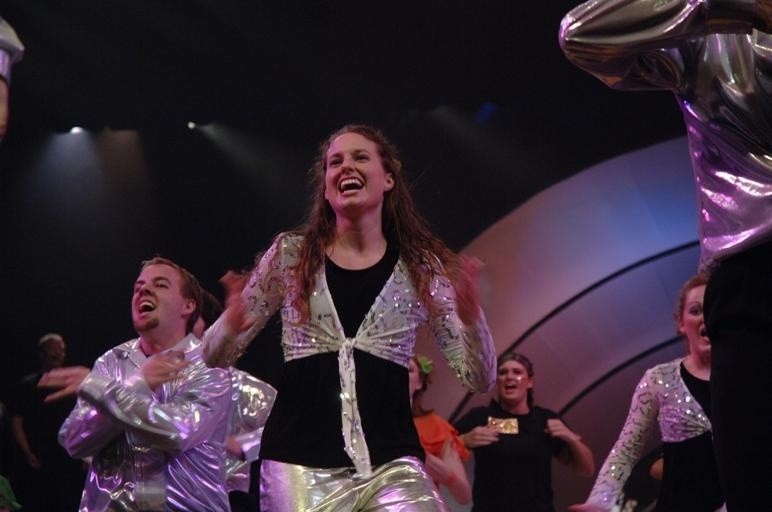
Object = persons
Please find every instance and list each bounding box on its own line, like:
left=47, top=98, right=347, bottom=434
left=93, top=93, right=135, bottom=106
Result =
left=36, top=257, right=232, bottom=512
left=190, top=290, right=278, bottom=512
left=14, top=332, right=83, bottom=512
left=566, top=272, right=728, bottom=512
left=450, top=350, right=598, bottom=512
left=201, top=122, right=498, bottom=512
left=555, top=0, right=772, bottom=511
left=408, top=355, right=474, bottom=512
